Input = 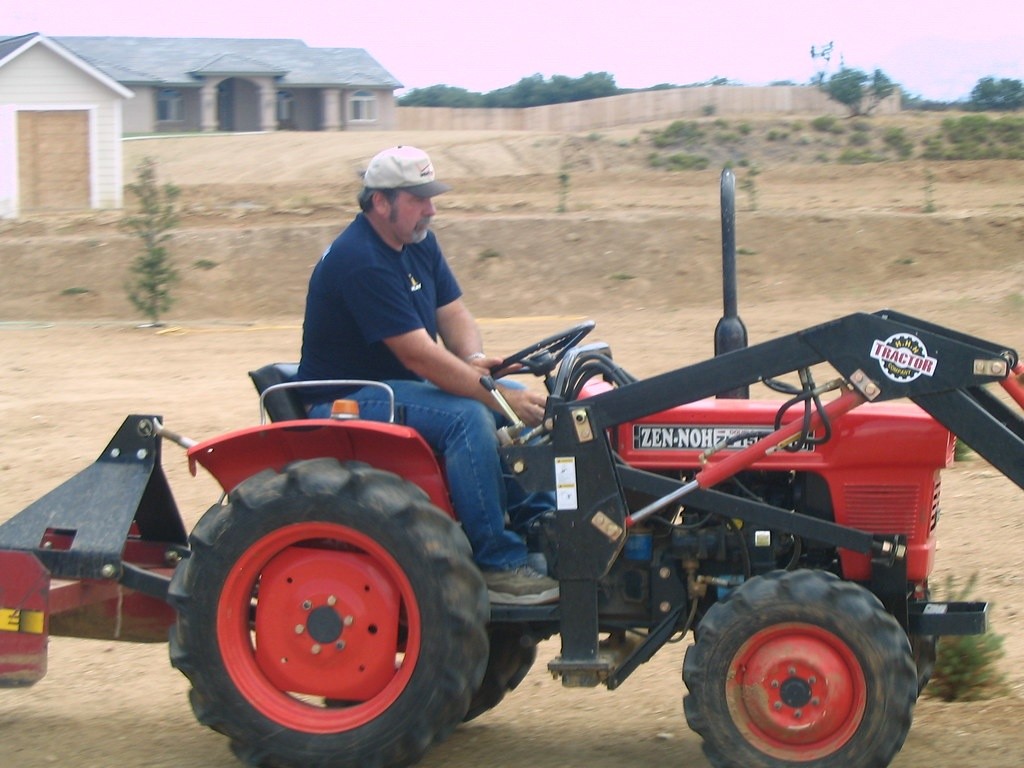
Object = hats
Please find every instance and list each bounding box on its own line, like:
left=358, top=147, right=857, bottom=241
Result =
left=363, top=145, right=452, bottom=198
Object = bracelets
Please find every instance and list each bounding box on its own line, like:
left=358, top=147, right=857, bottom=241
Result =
left=467, top=353, right=485, bottom=363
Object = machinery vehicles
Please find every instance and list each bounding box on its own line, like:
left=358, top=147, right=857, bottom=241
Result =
left=0, top=168, right=1024, bottom=768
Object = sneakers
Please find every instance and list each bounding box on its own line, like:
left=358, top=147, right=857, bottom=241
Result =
left=479, top=565, right=560, bottom=606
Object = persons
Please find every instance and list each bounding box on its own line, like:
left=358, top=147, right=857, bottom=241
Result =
left=294, top=146, right=560, bottom=607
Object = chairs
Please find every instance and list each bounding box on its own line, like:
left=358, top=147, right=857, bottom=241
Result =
left=248, top=360, right=308, bottom=426
